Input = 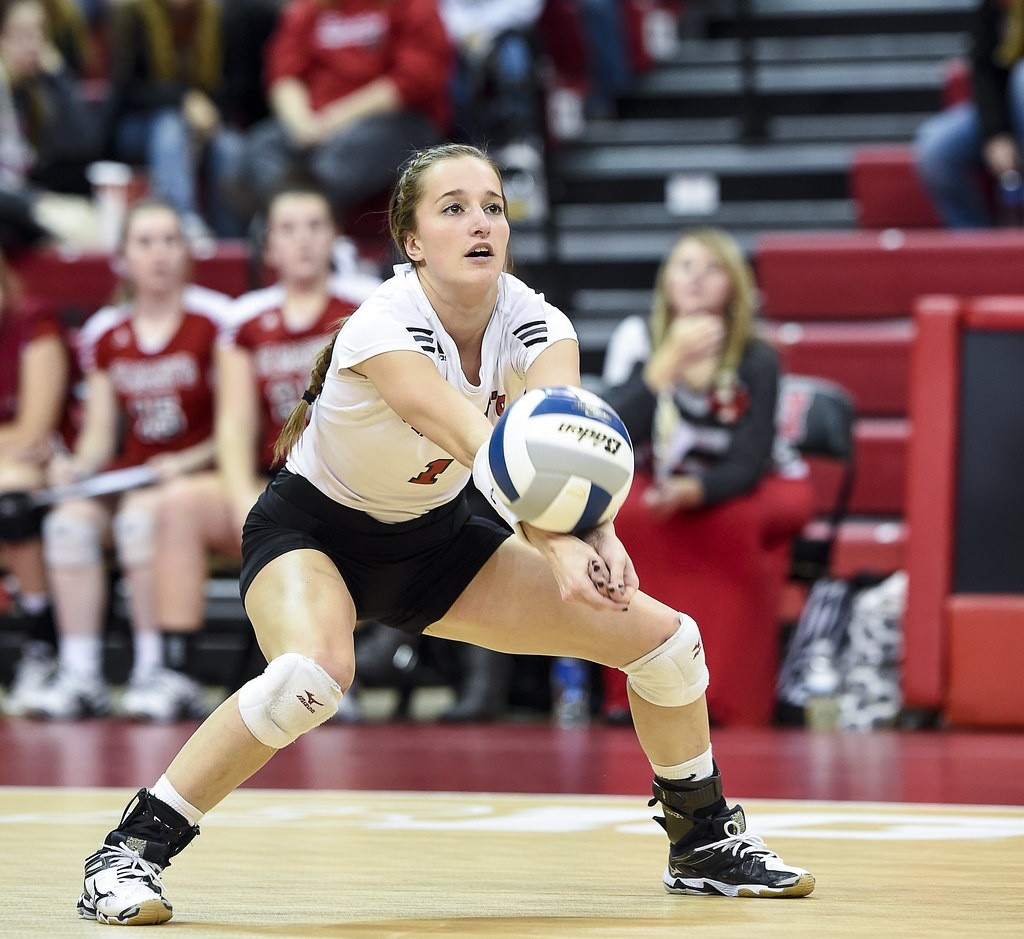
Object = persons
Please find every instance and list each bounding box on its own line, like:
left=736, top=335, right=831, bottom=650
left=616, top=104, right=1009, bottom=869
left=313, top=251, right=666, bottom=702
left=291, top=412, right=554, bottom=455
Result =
left=914, top=0, right=1024, bottom=233
left=75, top=142, right=815, bottom=924
left=0, top=0, right=784, bottom=729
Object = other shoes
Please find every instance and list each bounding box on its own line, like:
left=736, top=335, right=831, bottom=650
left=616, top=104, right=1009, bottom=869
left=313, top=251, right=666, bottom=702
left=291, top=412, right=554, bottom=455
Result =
left=109, top=669, right=149, bottom=718
left=123, top=664, right=208, bottom=720
left=2, top=659, right=57, bottom=715
left=26, top=667, right=111, bottom=718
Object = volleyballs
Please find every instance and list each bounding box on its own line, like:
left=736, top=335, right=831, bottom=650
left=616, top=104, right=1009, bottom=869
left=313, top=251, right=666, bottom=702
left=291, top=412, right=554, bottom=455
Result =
left=486, top=384, right=634, bottom=534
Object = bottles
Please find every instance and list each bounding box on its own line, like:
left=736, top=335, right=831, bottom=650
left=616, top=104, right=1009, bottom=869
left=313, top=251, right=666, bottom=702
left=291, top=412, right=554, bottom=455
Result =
left=807, top=642, right=837, bottom=734
left=554, top=658, right=586, bottom=732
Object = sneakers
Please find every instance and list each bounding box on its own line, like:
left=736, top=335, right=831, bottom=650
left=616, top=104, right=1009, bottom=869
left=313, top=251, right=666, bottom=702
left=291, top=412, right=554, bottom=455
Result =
left=77, top=829, right=175, bottom=925
left=653, top=802, right=816, bottom=898
